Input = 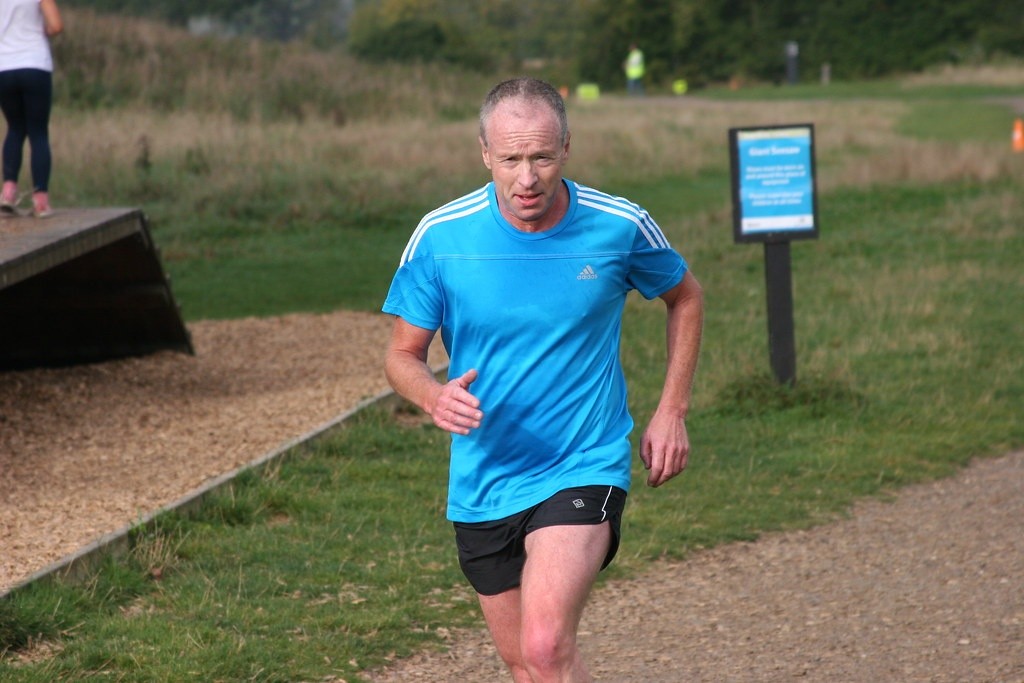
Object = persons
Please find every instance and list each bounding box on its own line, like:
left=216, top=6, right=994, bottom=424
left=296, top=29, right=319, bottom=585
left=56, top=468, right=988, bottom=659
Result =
left=623, top=43, right=645, bottom=95
left=381, top=80, right=705, bottom=683
left=0, top=0, right=62, bottom=217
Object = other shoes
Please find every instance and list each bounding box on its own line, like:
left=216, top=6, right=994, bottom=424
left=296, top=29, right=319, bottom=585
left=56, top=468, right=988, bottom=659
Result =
left=32, top=192, right=53, bottom=218
left=0, top=181, right=17, bottom=211
